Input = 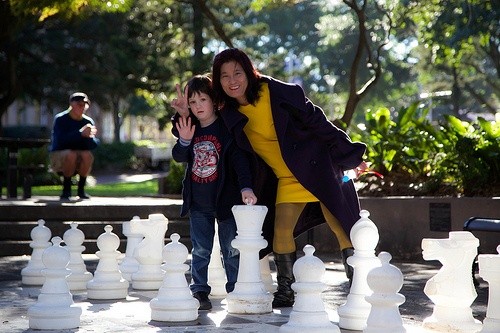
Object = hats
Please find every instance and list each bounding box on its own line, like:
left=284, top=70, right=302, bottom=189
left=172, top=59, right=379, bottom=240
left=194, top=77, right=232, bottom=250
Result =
left=70, top=92, right=90, bottom=106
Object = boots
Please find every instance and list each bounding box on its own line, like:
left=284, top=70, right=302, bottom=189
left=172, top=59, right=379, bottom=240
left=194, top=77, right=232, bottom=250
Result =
left=271, top=252, right=297, bottom=307
left=341, top=247, right=354, bottom=288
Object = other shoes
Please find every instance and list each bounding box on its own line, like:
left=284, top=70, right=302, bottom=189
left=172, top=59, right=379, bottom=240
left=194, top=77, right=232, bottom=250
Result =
left=226, top=281, right=236, bottom=293
left=60, top=190, right=71, bottom=199
left=193, top=291, right=212, bottom=311
left=77, top=190, right=89, bottom=198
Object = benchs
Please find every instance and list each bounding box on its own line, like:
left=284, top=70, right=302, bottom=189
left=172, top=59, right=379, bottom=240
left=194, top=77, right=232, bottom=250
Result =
left=0, top=139, right=78, bottom=199
left=463, top=216, right=500, bottom=287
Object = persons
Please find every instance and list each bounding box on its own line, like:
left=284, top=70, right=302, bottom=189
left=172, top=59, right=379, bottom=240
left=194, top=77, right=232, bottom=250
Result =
left=48, top=92, right=100, bottom=203
left=172, top=74, right=257, bottom=310
left=171, top=47, right=369, bottom=309
